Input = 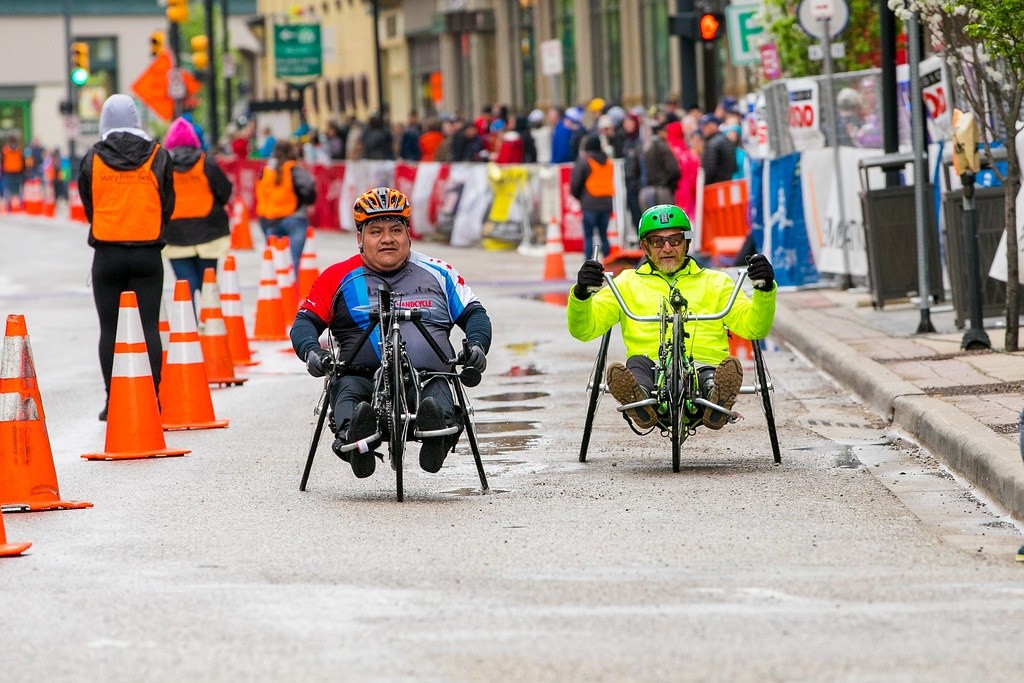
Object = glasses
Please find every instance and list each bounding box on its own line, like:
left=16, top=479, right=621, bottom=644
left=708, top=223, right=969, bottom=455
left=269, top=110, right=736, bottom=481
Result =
left=646, top=233, right=684, bottom=248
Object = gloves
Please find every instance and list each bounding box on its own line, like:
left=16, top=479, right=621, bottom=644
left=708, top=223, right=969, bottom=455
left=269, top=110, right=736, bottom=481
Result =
left=458, top=345, right=487, bottom=375
left=574, top=260, right=605, bottom=300
left=305, top=348, right=335, bottom=378
left=745, top=253, right=774, bottom=292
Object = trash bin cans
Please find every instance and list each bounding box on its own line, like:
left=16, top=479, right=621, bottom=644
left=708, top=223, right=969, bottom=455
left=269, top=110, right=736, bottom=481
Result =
left=940, top=147, right=1024, bottom=330
left=855, top=150, right=945, bottom=309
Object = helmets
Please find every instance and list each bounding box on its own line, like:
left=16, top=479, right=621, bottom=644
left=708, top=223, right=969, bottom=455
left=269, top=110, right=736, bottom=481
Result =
left=638, top=204, right=691, bottom=236
left=353, top=187, right=410, bottom=231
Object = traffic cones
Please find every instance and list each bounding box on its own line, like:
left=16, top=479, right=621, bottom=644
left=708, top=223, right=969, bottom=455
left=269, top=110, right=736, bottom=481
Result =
left=158, top=279, right=230, bottom=431
left=68, top=182, right=90, bottom=224
left=1, top=176, right=55, bottom=218
left=248, top=225, right=321, bottom=340
left=0, top=313, right=94, bottom=513
left=543, top=215, right=566, bottom=282
left=606, top=210, right=620, bottom=256
left=225, top=198, right=255, bottom=251
left=81, top=291, right=192, bottom=462
left=196, top=256, right=259, bottom=383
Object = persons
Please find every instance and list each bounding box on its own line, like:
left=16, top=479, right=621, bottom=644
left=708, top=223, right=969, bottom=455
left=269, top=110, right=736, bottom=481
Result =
left=0, top=95, right=776, bottom=431
left=289, top=185, right=492, bottom=480
left=834, top=76, right=889, bottom=150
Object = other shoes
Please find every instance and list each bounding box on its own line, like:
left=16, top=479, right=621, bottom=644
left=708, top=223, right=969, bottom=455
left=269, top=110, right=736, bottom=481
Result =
left=605, top=362, right=660, bottom=429
left=416, top=396, right=450, bottom=472
left=702, top=356, right=744, bottom=431
left=346, top=402, right=377, bottom=479
left=99, top=403, right=109, bottom=421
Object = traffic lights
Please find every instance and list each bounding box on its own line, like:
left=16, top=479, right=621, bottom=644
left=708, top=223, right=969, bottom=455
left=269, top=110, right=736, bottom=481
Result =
left=695, top=11, right=725, bottom=41
left=166, top=0, right=187, bottom=21
left=150, top=33, right=166, bottom=60
left=70, top=43, right=90, bottom=83
left=191, top=35, right=208, bottom=70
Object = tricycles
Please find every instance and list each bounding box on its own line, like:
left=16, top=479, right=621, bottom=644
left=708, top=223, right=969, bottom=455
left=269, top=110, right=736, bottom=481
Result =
left=578, top=244, right=782, bottom=472
left=299, top=284, right=489, bottom=501
